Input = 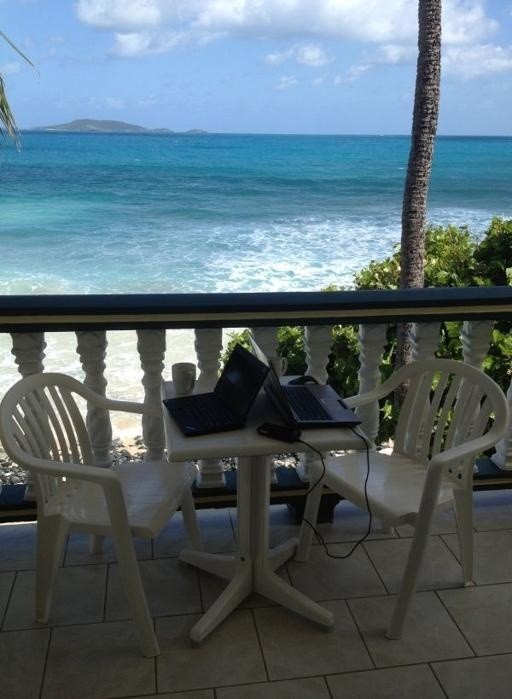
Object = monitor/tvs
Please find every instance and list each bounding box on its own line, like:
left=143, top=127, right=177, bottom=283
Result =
left=247, top=333, right=362, bottom=429
left=162, top=343, right=270, bottom=437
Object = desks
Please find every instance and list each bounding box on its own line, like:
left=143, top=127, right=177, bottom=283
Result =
left=159, top=376, right=374, bottom=641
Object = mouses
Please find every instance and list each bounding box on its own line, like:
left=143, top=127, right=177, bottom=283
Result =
left=288, top=376, right=318, bottom=385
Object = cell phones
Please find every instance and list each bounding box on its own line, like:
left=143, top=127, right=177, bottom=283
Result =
left=256, top=423, right=300, bottom=444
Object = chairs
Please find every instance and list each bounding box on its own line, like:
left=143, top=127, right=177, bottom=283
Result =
left=294, top=358, right=510, bottom=643
left=0, top=371, right=200, bottom=659
